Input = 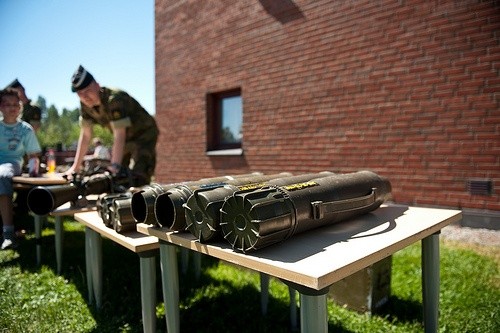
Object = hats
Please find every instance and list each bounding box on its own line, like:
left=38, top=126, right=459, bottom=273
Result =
left=72, top=65, right=94, bottom=92
left=5, top=79, right=23, bottom=89
left=92, top=137, right=103, bottom=143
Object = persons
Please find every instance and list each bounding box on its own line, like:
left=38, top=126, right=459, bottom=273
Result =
left=90, top=138, right=111, bottom=161
left=65, top=64, right=159, bottom=189
left=0, top=88, right=43, bottom=249
left=8, top=79, right=43, bottom=171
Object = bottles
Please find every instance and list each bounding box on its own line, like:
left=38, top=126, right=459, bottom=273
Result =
left=47, top=149, right=56, bottom=173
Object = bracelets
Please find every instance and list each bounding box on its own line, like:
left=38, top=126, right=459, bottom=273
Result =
left=111, top=163, right=121, bottom=168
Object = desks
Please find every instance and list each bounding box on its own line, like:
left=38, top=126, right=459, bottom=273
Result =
left=13, top=172, right=464, bottom=333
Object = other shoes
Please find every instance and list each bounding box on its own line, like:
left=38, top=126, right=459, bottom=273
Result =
left=2, top=228, right=18, bottom=250
